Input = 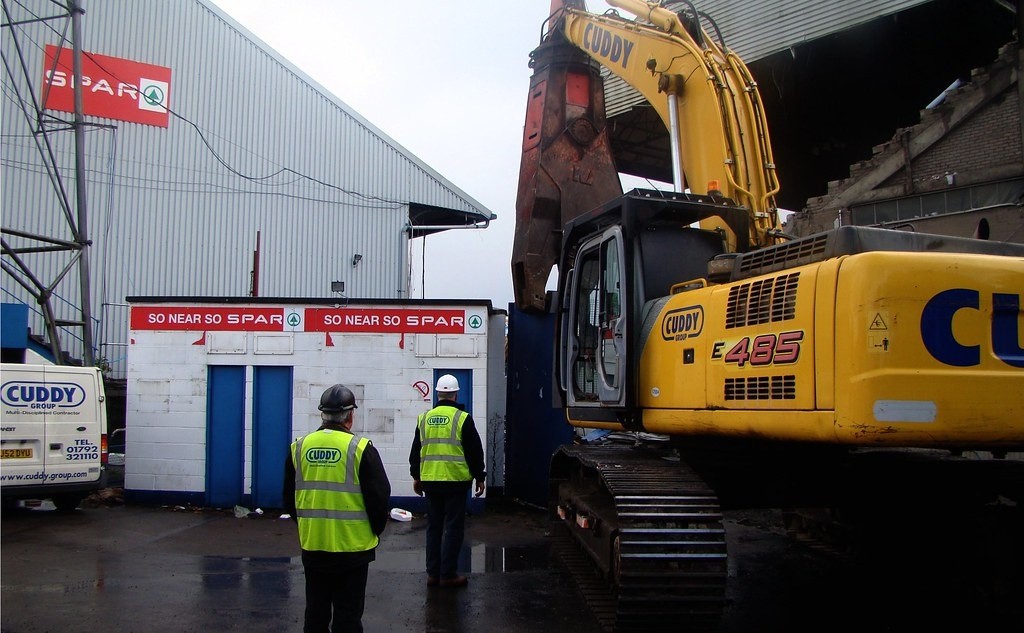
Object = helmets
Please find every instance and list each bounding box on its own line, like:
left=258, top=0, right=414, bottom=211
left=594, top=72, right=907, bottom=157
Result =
left=318, top=384, right=358, bottom=412
left=435, top=374, right=460, bottom=392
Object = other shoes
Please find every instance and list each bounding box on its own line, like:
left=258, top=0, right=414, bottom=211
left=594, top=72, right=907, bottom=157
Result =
left=427, top=575, right=467, bottom=588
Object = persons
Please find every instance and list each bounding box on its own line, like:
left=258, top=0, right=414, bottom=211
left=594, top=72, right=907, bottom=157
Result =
left=282, top=384, right=393, bottom=633
left=409, top=374, right=490, bottom=592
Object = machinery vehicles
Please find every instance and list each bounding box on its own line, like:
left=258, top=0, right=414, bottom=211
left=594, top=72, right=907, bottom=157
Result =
left=511, top=0, right=1024, bottom=633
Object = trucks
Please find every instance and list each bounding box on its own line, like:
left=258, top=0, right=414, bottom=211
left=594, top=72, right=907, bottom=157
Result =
left=0, top=363, right=108, bottom=511
